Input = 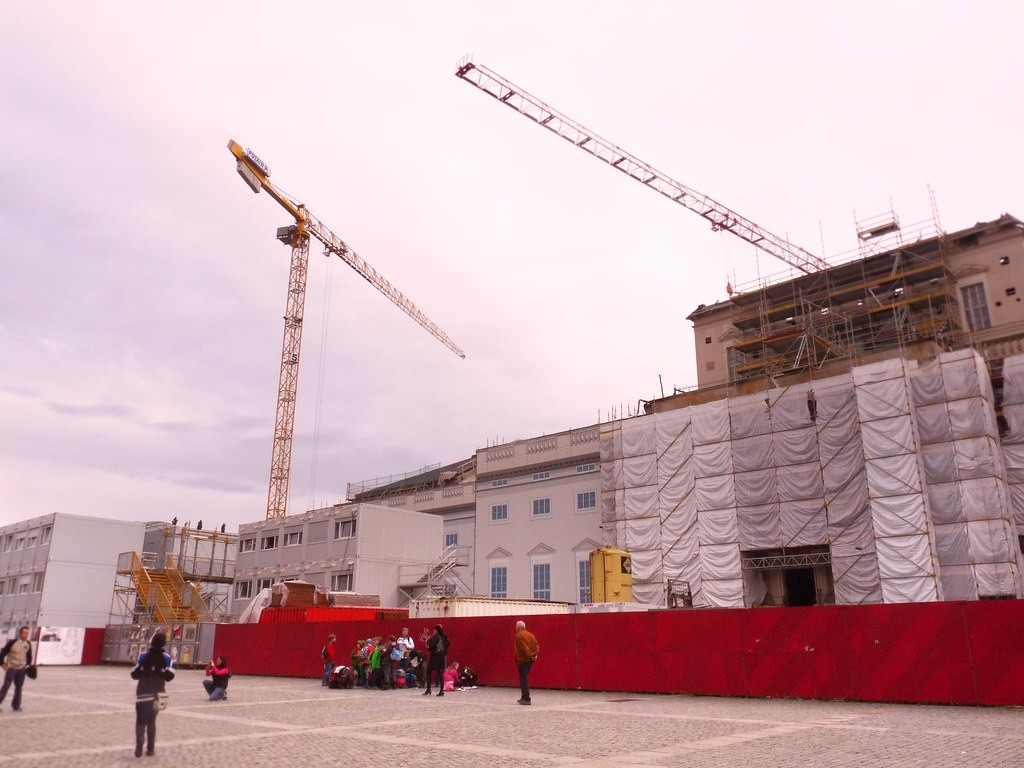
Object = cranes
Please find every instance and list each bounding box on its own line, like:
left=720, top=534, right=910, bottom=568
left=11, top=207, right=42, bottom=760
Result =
left=454, top=52, right=831, bottom=274
left=227, top=138, right=467, bottom=518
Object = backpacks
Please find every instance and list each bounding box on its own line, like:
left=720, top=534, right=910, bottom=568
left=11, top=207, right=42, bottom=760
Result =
left=322, top=646, right=326, bottom=658
left=435, top=634, right=446, bottom=656
left=390, top=648, right=402, bottom=660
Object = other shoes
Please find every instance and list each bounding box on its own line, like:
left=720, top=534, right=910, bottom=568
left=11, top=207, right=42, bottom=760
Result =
left=12, top=703, right=19, bottom=710
left=222, top=691, right=227, bottom=699
left=146, top=750, right=154, bottom=756
left=518, top=698, right=531, bottom=705
left=135, top=750, right=142, bottom=757
left=322, top=681, right=329, bottom=686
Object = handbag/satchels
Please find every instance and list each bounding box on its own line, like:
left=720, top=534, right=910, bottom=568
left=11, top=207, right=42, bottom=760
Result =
left=153, top=693, right=168, bottom=711
left=443, top=681, right=454, bottom=690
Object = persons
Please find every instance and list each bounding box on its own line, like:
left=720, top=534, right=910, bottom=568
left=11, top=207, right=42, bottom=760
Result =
left=350, top=628, right=427, bottom=690
left=329, top=665, right=357, bottom=689
left=515, top=621, right=539, bottom=705
left=172, top=517, right=226, bottom=533
left=0, top=626, right=33, bottom=711
left=444, top=662, right=478, bottom=691
left=423, top=624, right=450, bottom=696
left=322, top=634, right=337, bottom=686
left=131, top=632, right=175, bottom=758
left=203, top=656, right=230, bottom=701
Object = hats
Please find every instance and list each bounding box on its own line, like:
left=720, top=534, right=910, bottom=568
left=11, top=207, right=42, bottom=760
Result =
left=151, top=632, right=166, bottom=647
left=27, top=666, right=36, bottom=678
left=366, top=638, right=372, bottom=644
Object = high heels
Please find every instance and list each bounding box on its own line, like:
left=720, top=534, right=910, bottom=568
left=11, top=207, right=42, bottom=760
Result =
left=436, top=690, right=444, bottom=697
left=422, top=688, right=432, bottom=695
